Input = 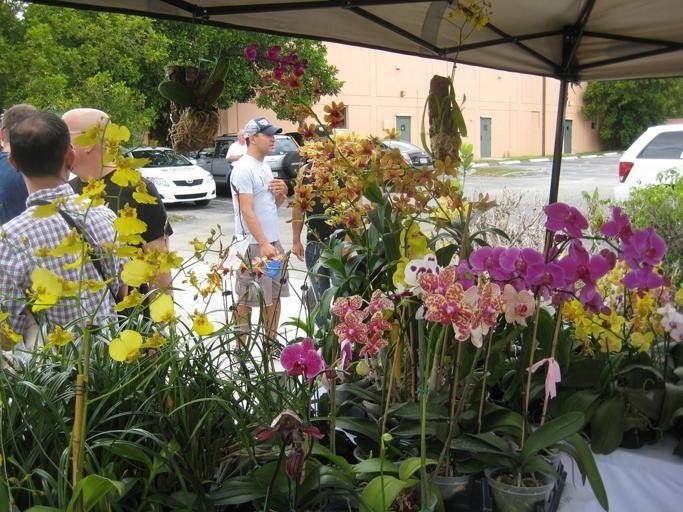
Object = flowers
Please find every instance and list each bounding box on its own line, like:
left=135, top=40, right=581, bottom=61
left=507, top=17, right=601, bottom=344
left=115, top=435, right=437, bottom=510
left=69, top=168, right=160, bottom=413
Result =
left=457, top=201, right=666, bottom=480
left=406, top=252, right=499, bottom=479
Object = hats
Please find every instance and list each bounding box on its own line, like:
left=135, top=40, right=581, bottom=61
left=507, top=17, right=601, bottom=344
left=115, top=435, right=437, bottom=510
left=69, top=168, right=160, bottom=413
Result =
left=243, top=116, right=282, bottom=140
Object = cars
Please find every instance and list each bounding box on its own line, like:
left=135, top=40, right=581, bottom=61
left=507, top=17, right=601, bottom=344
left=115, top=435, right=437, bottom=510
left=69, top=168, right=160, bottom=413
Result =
left=125, top=146, right=217, bottom=210
left=372, top=138, right=436, bottom=178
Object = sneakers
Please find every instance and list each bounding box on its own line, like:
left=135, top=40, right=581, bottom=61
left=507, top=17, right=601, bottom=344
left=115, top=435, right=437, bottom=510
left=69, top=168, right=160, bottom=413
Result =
left=232, top=348, right=255, bottom=373
left=264, top=341, right=284, bottom=359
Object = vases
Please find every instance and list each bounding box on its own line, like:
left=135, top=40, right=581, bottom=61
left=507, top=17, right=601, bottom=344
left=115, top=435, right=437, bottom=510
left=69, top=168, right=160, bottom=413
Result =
left=484, top=464, right=555, bottom=511
left=433, top=475, right=468, bottom=500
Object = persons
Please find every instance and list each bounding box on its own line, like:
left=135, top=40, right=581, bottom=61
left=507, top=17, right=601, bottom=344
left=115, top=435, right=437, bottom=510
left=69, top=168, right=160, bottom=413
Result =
left=0, top=103, right=174, bottom=359
left=291, top=125, right=353, bottom=328
left=226, top=128, right=248, bottom=170
left=229, top=116, right=289, bottom=374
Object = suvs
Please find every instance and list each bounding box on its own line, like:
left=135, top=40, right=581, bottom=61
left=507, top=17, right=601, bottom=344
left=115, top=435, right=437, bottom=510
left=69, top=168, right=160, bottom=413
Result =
left=615, top=122, right=683, bottom=211
left=191, top=132, right=314, bottom=198
